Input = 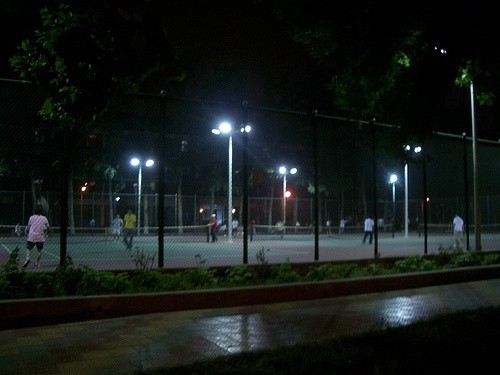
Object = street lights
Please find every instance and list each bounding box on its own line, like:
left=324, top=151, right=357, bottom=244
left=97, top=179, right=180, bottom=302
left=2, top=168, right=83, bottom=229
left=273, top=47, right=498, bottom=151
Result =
left=212, top=122, right=252, bottom=243
left=404, top=145, right=422, bottom=237
left=388, top=174, right=398, bottom=237
left=279, top=166, right=297, bottom=235
left=131, top=157, right=154, bottom=237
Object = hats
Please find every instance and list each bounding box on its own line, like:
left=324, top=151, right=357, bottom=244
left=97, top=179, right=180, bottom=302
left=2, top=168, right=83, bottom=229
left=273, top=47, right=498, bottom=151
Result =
left=212, top=214, right=216, bottom=216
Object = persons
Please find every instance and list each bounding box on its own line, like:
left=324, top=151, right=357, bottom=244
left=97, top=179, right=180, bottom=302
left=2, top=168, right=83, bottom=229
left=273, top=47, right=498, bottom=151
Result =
left=208, top=214, right=218, bottom=242
left=452, top=213, right=464, bottom=248
left=111, top=214, right=123, bottom=239
left=326, top=219, right=332, bottom=234
left=275, top=219, right=283, bottom=235
left=23, top=205, right=49, bottom=267
left=232, top=218, right=239, bottom=235
left=15, top=221, right=23, bottom=238
left=296, top=221, right=300, bottom=232
left=362, top=215, right=374, bottom=244
left=122, top=208, right=137, bottom=251
left=90, top=216, right=96, bottom=235
left=339, top=218, right=347, bottom=235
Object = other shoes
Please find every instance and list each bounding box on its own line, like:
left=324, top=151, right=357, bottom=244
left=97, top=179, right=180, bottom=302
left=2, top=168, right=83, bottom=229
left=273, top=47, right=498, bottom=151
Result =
left=23, top=258, right=30, bottom=268
left=34, top=264, right=40, bottom=268
